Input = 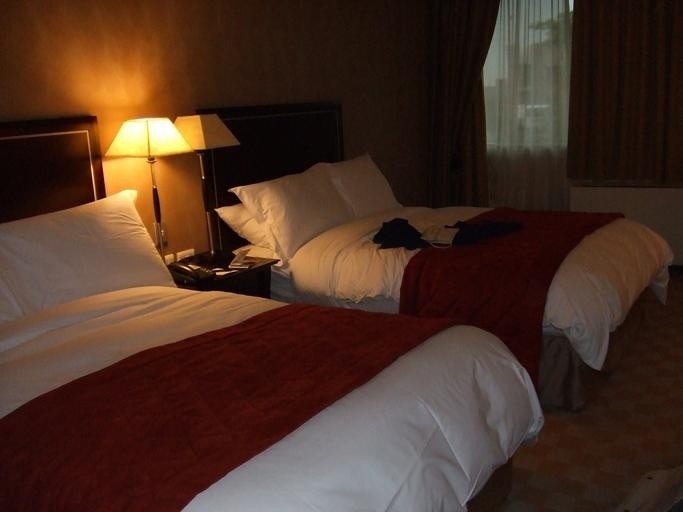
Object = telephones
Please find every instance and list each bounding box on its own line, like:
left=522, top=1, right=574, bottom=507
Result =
left=167, top=262, right=215, bottom=286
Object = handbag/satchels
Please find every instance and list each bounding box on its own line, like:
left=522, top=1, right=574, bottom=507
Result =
left=420, top=225, right=460, bottom=248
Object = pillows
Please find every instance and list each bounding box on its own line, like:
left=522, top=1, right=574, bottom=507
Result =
left=214, top=204, right=269, bottom=248
left=228, top=163, right=354, bottom=267
left=325, top=153, right=403, bottom=217
left=0, top=191, right=177, bottom=316
left=0, top=279, right=24, bottom=326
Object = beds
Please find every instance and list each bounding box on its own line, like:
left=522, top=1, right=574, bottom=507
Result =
left=0, top=115, right=544, bottom=511
left=196, top=100, right=647, bottom=412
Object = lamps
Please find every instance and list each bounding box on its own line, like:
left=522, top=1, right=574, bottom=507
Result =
left=174, top=114, right=240, bottom=265
left=105, top=117, right=194, bottom=265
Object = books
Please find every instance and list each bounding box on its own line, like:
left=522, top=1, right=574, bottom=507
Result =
left=228, top=248, right=256, bottom=269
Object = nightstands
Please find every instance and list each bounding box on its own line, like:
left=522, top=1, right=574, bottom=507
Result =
left=167, top=252, right=281, bottom=299
